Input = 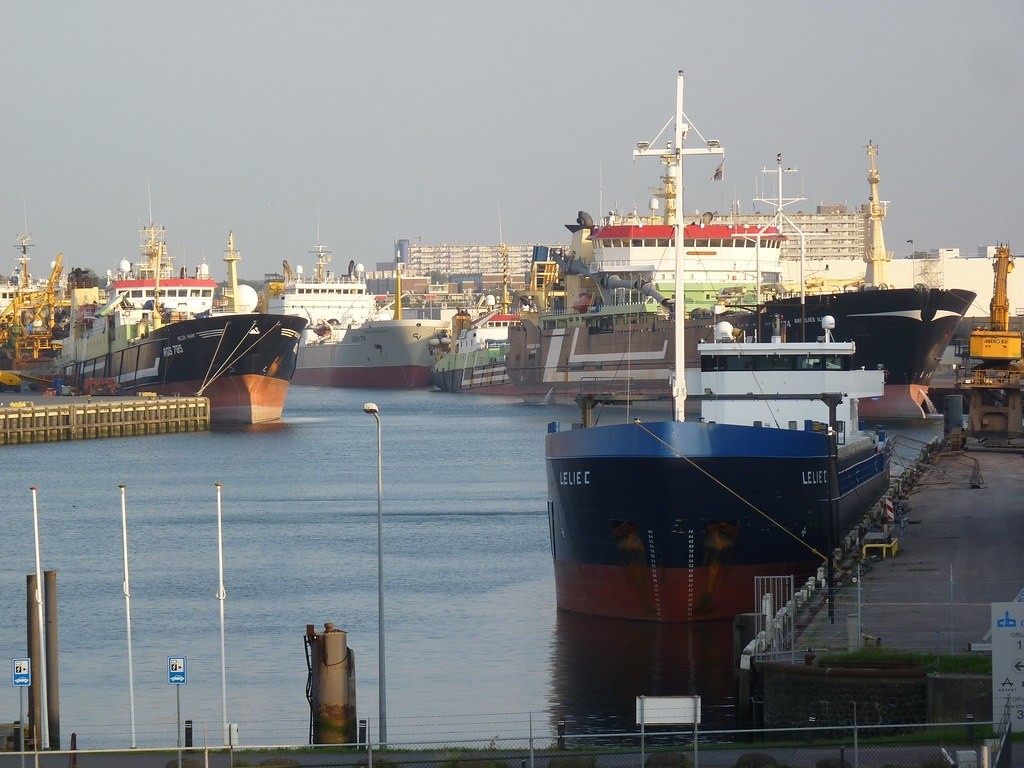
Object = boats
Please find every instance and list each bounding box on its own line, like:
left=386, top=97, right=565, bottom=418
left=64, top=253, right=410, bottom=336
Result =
left=503, top=150, right=979, bottom=423
left=260, top=244, right=451, bottom=389
left=545, top=68, right=841, bottom=630
left=52, top=223, right=308, bottom=426
left=429, top=244, right=525, bottom=397
left=1, top=231, right=70, bottom=396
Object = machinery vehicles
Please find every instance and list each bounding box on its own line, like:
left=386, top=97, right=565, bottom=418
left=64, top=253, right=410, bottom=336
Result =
left=942, top=243, right=1024, bottom=457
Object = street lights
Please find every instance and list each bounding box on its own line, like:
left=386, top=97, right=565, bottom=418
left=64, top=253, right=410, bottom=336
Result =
left=362, top=403, right=388, bottom=750
